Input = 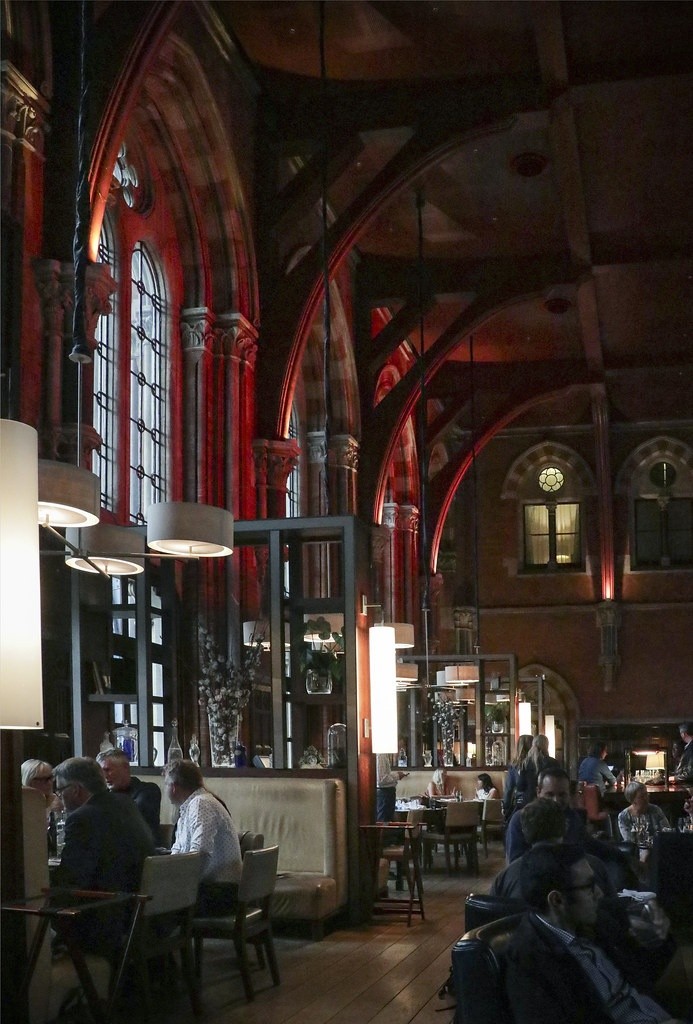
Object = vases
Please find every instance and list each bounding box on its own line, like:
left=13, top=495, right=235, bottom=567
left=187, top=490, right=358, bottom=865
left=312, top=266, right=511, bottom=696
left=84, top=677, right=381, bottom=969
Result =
left=207, top=713, right=241, bottom=766
left=441, top=726, right=454, bottom=766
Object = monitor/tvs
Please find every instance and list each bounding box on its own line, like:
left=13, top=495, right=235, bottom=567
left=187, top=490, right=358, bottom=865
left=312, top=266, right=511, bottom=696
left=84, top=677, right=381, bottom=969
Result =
left=250, top=754, right=265, bottom=768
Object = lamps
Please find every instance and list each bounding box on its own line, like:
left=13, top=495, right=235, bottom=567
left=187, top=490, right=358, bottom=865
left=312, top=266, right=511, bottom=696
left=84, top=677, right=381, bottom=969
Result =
left=545, top=710, right=555, bottom=759
left=395, top=181, right=481, bottom=692
left=437, top=336, right=511, bottom=704
left=517, top=689, right=532, bottom=735
left=645, top=752, right=667, bottom=783
left=0, top=417, right=46, bottom=731
left=361, top=594, right=399, bottom=754
left=40, top=0, right=234, bottom=576
left=244, top=0, right=415, bottom=650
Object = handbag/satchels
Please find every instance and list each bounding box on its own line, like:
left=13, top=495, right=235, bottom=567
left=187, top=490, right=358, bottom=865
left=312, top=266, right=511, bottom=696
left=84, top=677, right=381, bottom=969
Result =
left=504, top=787, right=529, bottom=824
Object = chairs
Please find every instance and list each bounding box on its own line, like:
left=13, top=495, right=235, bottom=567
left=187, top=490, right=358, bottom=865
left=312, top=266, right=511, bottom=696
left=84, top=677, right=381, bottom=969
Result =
left=193, top=845, right=280, bottom=1002
left=379, top=810, right=424, bottom=896
left=478, top=799, right=504, bottom=859
left=423, top=801, right=481, bottom=875
left=60, top=854, right=205, bottom=1024
left=237, top=829, right=264, bottom=861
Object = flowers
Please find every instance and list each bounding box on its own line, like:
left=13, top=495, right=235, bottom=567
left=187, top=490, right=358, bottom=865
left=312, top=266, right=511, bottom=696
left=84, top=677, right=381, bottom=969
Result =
left=422, top=693, right=466, bottom=725
left=194, top=597, right=269, bottom=712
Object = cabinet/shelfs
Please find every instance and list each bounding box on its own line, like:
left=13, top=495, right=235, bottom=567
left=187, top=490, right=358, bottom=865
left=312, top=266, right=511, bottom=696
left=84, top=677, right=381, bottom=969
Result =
left=78, top=514, right=388, bottom=777
left=485, top=674, right=546, bottom=736
left=396, top=654, right=519, bottom=769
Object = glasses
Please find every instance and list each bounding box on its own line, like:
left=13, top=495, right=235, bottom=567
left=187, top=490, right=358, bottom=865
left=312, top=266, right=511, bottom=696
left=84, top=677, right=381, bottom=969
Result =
left=33, top=775, right=55, bottom=782
left=572, top=881, right=597, bottom=893
left=53, top=784, right=73, bottom=797
left=635, top=792, right=650, bottom=800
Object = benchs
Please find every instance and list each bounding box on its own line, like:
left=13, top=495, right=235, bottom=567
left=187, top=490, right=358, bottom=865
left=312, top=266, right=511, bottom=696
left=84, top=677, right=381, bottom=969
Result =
left=130, top=773, right=346, bottom=939
left=21, top=785, right=112, bottom=1024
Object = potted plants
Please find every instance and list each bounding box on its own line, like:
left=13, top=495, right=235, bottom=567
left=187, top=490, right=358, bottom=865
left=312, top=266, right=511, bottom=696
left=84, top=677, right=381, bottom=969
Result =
left=486, top=702, right=509, bottom=733
left=294, top=617, right=346, bottom=694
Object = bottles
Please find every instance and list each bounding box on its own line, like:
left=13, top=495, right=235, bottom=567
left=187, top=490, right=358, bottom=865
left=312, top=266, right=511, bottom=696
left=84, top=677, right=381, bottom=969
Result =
left=46, top=811, right=57, bottom=859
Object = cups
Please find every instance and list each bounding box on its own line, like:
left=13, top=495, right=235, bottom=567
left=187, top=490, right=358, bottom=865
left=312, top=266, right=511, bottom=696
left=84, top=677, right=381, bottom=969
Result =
left=678, top=818, right=685, bottom=832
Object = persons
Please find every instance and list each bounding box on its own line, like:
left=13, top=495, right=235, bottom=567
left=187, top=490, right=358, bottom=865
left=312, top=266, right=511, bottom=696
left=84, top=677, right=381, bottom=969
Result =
left=375, top=754, right=405, bottom=879
left=20, top=748, right=242, bottom=1024
left=472, top=722, right=693, bottom=1023
left=419, top=768, right=455, bottom=833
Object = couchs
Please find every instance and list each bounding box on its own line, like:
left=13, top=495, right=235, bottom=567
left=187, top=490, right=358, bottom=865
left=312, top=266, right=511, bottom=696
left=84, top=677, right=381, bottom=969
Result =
left=452, top=831, right=693, bottom=1024
left=392, top=770, right=510, bottom=802
